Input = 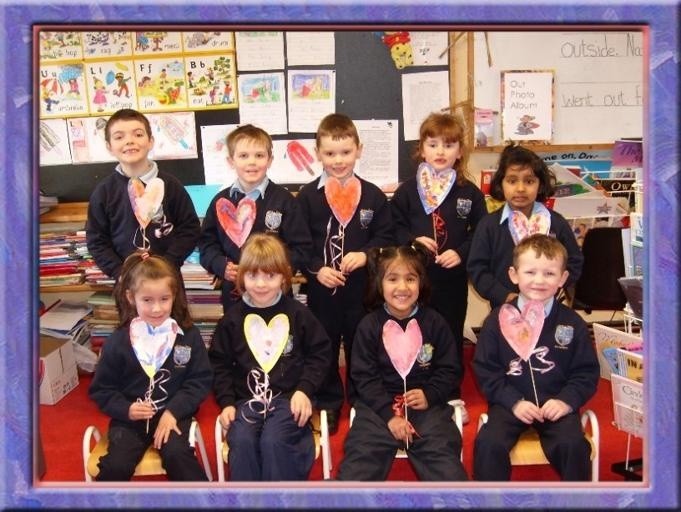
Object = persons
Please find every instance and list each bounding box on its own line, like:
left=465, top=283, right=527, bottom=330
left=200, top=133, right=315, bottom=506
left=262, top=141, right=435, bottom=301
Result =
left=90, top=245, right=217, bottom=481
left=386, top=110, right=491, bottom=426
left=84, top=109, right=202, bottom=325
left=200, top=124, right=316, bottom=318
left=466, top=145, right=585, bottom=311
left=336, top=239, right=473, bottom=482
left=204, top=231, right=335, bottom=481
left=470, top=232, right=602, bottom=483
left=282, top=111, right=391, bottom=435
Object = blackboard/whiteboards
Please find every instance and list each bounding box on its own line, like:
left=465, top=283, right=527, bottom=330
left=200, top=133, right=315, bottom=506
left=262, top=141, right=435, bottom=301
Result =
left=37, top=25, right=458, bottom=223
left=465, top=26, right=643, bottom=152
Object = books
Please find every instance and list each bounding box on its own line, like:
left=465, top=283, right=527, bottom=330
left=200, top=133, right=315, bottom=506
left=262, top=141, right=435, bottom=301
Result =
left=483, top=193, right=506, bottom=214
left=546, top=137, right=644, bottom=441
left=38, top=220, right=306, bottom=360
left=38, top=194, right=59, bottom=214
left=474, top=108, right=502, bottom=148
left=481, top=169, right=498, bottom=199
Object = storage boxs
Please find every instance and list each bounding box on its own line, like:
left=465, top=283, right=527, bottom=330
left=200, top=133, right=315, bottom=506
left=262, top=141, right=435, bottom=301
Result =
left=37, top=334, right=81, bottom=407
